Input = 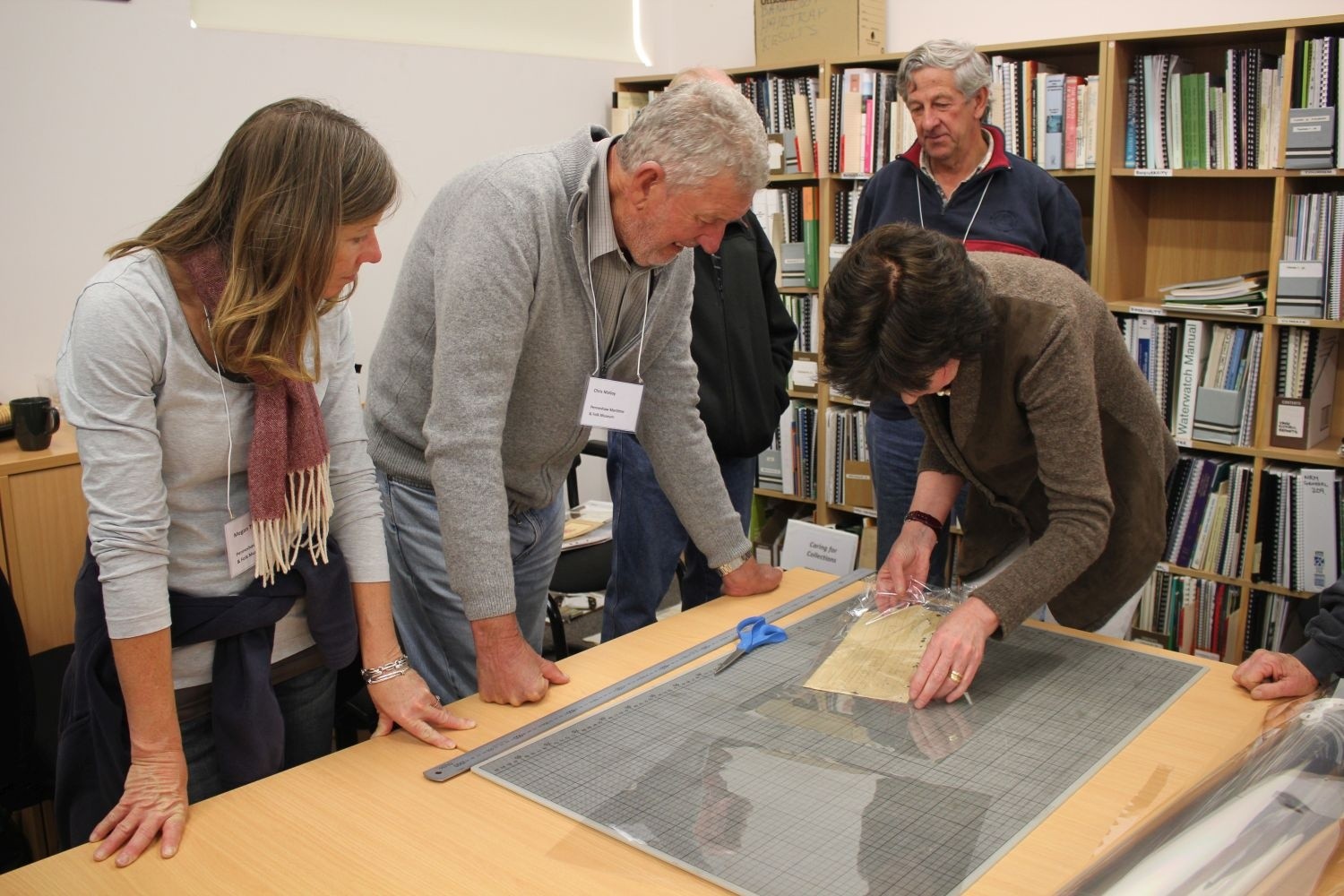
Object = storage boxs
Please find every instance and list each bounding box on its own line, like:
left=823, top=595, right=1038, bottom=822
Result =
left=754, top=0, right=886, bottom=66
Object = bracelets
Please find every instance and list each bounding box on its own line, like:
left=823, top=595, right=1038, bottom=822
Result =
left=360, top=654, right=411, bottom=684
left=714, top=550, right=752, bottom=578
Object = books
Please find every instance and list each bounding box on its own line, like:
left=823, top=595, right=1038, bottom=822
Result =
left=781, top=517, right=860, bottom=576
left=610, top=76, right=816, bottom=572
left=1118, top=38, right=1344, bottom=665
left=561, top=501, right=613, bottom=552
left=826, top=55, right=1101, bottom=588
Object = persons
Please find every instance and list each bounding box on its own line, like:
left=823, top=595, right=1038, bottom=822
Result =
left=1233, top=577, right=1344, bottom=702
left=53, top=96, right=479, bottom=870
left=362, top=81, right=784, bottom=710
left=851, top=38, right=1088, bottom=589
left=600, top=66, right=798, bottom=646
left=821, top=224, right=1181, bottom=710
left=588, top=681, right=990, bottom=895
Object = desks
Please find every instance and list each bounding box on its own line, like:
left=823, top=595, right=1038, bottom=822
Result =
left=0, top=568, right=1324, bottom=896
left=826, top=34, right=1107, bottom=589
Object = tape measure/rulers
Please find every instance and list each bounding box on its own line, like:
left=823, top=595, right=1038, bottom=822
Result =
left=421, top=567, right=902, bottom=783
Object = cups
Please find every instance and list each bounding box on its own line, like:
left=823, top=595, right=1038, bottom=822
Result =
left=10, top=395, right=60, bottom=451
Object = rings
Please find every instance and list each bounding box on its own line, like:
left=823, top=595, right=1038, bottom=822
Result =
left=433, top=695, right=440, bottom=708
left=948, top=670, right=963, bottom=683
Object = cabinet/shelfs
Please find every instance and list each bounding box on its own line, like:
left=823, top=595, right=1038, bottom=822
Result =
left=1101, top=15, right=1344, bottom=666
left=613, top=59, right=824, bottom=566
left=0, top=416, right=92, bottom=657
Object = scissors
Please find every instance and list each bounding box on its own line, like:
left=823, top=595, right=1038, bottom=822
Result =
left=713, top=616, right=788, bottom=678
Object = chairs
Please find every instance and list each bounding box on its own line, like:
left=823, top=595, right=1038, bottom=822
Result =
left=541, top=439, right=613, bottom=657
